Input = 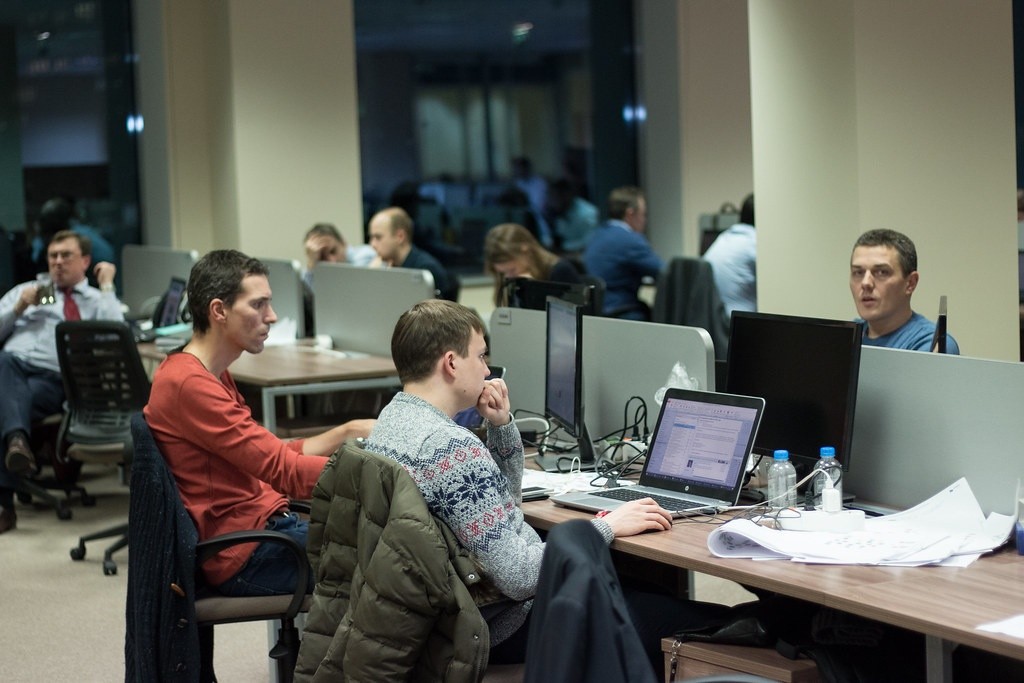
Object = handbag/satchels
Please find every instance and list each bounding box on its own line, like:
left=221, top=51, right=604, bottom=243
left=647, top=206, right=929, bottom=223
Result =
left=658, top=598, right=776, bottom=644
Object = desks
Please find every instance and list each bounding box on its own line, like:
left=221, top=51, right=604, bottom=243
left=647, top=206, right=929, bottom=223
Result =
left=136, top=341, right=396, bottom=442
left=523, top=446, right=1024, bottom=683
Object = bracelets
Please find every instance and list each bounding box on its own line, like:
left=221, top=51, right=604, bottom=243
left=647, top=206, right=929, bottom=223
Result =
left=101, top=282, right=113, bottom=289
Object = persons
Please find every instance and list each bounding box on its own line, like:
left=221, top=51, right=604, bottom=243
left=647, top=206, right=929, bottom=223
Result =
left=547, top=180, right=599, bottom=250
left=300, top=223, right=378, bottom=298
left=366, top=297, right=672, bottom=666
left=482, top=223, right=578, bottom=310
left=143, top=249, right=376, bottom=597
left=0, top=201, right=126, bottom=535
left=498, top=154, right=563, bottom=252
left=366, top=206, right=459, bottom=303
left=581, top=184, right=666, bottom=319
left=700, top=193, right=757, bottom=315
left=849, top=229, right=961, bottom=354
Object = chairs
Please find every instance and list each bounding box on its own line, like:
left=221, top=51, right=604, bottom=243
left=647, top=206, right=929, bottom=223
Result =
left=667, top=256, right=729, bottom=360
left=55, top=321, right=151, bottom=574
left=342, top=439, right=526, bottom=682
left=548, top=518, right=661, bottom=683
left=129, top=412, right=309, bottom=683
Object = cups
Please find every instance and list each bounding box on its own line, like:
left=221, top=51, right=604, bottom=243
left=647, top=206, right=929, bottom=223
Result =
left=753, top=455, right=774, bottom=486
left=33, top=275, right=55, bottom=304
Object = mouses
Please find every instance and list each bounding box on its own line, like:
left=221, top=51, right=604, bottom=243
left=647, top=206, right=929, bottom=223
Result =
left=638, top=516, right=673, bottom=535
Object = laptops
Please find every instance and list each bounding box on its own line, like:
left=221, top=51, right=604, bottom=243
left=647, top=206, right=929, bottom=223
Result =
left=450, top=366, right=507, bottom=429
left=134, top=277, right=187, bottom=344
left=548, top=387, right=766, bottom=519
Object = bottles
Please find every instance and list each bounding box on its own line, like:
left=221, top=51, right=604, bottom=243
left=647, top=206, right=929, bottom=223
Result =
left=768, top=451, right=798, bottom=511
left=813, top=447, right=842, bottom=510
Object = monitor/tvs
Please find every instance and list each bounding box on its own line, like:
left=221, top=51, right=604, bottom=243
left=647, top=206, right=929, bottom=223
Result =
left=507, top=278, right=597, bottom=317
left=534, top=295, right=604, bottom=471
left=724, top=310, right=863, bottom=507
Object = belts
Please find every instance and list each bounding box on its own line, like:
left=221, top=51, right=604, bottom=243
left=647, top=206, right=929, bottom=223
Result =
left=267, top=510, right=290, bottom=524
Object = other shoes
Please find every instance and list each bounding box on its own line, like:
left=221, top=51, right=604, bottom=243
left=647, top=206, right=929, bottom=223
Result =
left=0, top=505, right=17, bottom=534
left=6, top=435, right=37, bottom=476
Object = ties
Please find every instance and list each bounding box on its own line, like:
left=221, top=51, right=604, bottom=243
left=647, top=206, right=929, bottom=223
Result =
left=59, top=285, right=81, bottom=323
